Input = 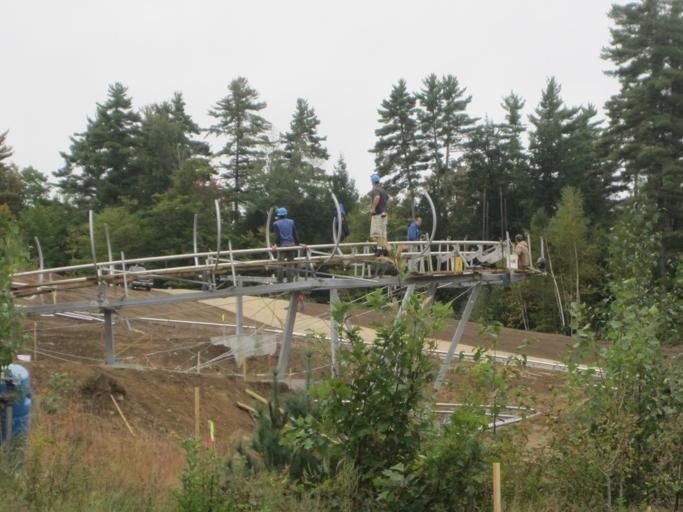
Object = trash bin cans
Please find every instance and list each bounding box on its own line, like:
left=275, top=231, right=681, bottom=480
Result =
left=0, top=362, right=32, bottom=471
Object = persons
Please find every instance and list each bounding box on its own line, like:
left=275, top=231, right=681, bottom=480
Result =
left=333, top=201, right=351, bottom=244
left=406, top=214, right=424, bottom=274
left=367, top=173, right=392, bottom=256
left=512, top=234, right=547, bottom=276
left=270, top=206, right=301, bottom=283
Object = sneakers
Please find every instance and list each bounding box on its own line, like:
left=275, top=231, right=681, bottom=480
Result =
left=375, top=249, right=388, bottom=257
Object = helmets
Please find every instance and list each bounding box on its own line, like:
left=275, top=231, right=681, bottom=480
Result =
left=371, top=175, right=379, bottom=183
left=277, top=208, right=288, bottom=215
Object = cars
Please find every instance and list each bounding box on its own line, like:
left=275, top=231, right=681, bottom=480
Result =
left=128, top=266, right=153, bottom=290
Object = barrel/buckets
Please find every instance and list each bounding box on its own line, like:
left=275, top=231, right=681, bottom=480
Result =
left=0, top=362, right=31, bottom=436
left=451, top=256, right=462, bottom=271
left=507, top=254, right=519, bottom=270
